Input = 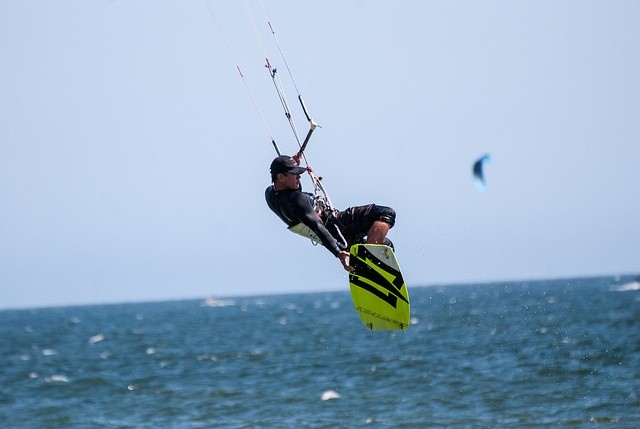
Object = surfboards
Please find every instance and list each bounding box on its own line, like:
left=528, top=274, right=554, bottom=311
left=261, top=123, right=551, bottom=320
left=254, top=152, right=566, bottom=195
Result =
left=349, top=244, right=409, bottom=331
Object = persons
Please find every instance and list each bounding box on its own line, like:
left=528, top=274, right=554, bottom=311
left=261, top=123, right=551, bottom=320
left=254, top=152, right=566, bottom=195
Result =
left=265, top=155, right=396, bottom=271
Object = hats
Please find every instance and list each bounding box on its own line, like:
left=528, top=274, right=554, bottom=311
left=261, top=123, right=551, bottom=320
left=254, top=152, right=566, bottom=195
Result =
left=270, top=155, right=307, bottom=174
